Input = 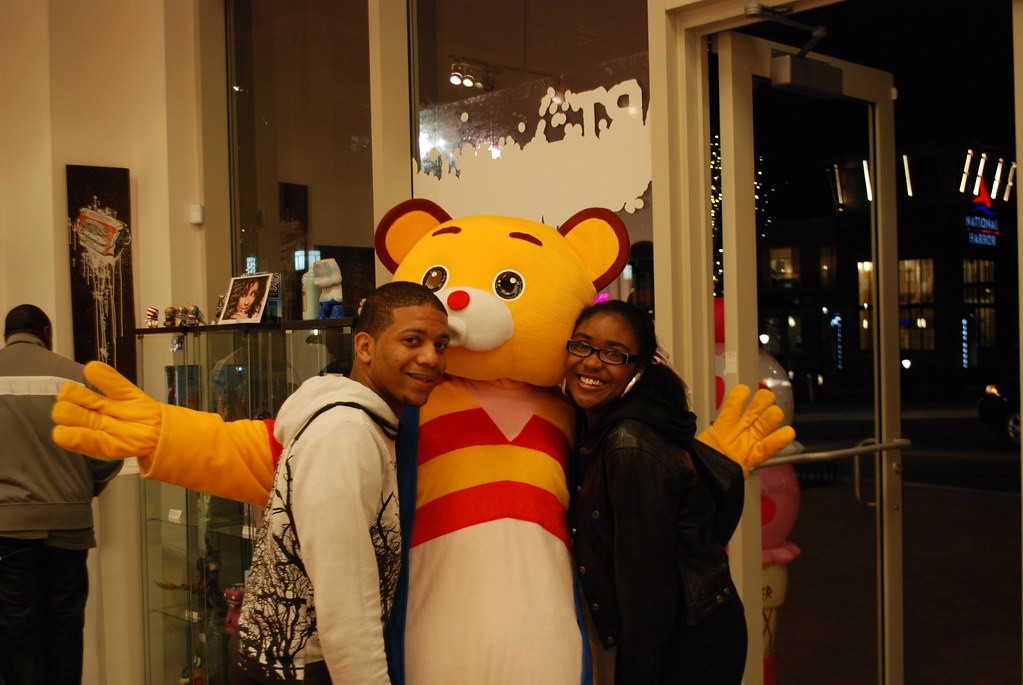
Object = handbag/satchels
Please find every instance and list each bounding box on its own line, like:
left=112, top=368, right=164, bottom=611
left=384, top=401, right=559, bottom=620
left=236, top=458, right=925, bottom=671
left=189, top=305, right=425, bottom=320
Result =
left=693, top=440, right=745, bottom=546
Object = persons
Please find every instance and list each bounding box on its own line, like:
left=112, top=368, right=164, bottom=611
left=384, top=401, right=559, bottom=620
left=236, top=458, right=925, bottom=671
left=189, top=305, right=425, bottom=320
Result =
left=565, top=301, right=751, bottom=684
left=222, top=276, right=270, bottom=320
left=232, top=283, right=449, bottom=685
left=1, top=303, right=124, bottom=685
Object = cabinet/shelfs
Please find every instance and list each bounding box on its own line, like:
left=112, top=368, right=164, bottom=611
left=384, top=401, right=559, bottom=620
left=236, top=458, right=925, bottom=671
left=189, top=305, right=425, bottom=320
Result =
left=134, top=315, right=353, bottom=685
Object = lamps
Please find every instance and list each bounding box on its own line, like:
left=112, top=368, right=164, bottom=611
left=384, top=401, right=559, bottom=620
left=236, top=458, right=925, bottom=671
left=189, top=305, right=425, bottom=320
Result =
left=450, top=57, right=496, bottom=93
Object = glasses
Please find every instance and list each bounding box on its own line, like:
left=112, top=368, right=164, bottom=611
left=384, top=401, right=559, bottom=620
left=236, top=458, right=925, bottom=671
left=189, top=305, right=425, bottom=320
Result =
left=566, top=340, right=639, bottom=365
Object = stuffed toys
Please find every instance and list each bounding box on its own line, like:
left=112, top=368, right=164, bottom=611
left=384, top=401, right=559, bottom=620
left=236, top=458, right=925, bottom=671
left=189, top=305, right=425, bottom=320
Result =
left=54, top=198, right=793, bottom=685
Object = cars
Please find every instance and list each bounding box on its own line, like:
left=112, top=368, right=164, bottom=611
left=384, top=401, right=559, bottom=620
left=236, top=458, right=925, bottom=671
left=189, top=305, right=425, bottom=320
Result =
left=976, top=365, right=1020, bottom=449
